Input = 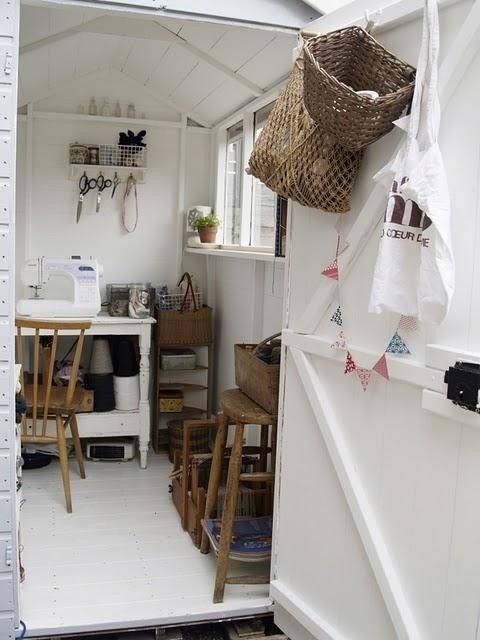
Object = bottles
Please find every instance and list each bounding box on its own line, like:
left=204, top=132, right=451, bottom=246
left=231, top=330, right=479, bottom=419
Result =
left=128, top=105, right=136, bottom=118
left=115, top=100, right=122, bottom=117
left=99, top=98, right=117, bottom=119
left=89, top=95, right=97, bottom=115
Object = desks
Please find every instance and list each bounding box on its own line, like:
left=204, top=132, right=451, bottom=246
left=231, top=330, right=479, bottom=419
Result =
left=15, top=307, right=156, bottom=468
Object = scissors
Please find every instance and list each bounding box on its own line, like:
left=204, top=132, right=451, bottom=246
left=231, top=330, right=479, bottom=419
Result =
left=77, top=175, right=96, bottom=224
left=96, top=175, right=112, bottom=213
left=112, top=177, right=121, bottom=198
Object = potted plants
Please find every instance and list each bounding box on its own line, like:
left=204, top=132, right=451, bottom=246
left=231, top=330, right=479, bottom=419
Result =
left=196, top=215, right=221, bottom=243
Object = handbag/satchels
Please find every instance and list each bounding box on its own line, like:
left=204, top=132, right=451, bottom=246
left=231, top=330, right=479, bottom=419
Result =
left=247, top=34, right=359, bottom=211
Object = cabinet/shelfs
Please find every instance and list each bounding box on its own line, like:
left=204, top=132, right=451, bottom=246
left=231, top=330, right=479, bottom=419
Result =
left=156, top=342, right=215, bottom=454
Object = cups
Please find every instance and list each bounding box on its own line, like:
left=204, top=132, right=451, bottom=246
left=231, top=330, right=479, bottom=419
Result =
left=89, top=339, right=114, bottom=374
left=111, top=340, right=138, bottom=376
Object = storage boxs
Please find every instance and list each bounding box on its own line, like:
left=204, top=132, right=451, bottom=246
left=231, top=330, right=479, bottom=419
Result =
left=106, top=282, right=156, bottom=316
left=160, top=390, right=183, bottom=412
left=161, top=349, right=197, bottom=370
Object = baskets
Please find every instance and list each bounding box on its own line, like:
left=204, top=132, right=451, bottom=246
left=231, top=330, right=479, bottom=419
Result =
left=153, top=271, right=213, bottom=345
left=98, top=143, right=147, bottom=167
left=303, top=25, right=415, bottom=151
left=155, top=284, right=204, bottom=311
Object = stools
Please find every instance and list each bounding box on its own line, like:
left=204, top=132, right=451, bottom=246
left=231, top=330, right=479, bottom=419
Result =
left=200, top=389, right=278, bottom=603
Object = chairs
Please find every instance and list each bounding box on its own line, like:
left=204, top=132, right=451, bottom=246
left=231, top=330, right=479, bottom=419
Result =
left=15, top=318, right=92, bottom=514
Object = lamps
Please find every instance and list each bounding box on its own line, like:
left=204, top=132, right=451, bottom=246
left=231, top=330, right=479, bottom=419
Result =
left=186, top=206, right=211, bottom=243
